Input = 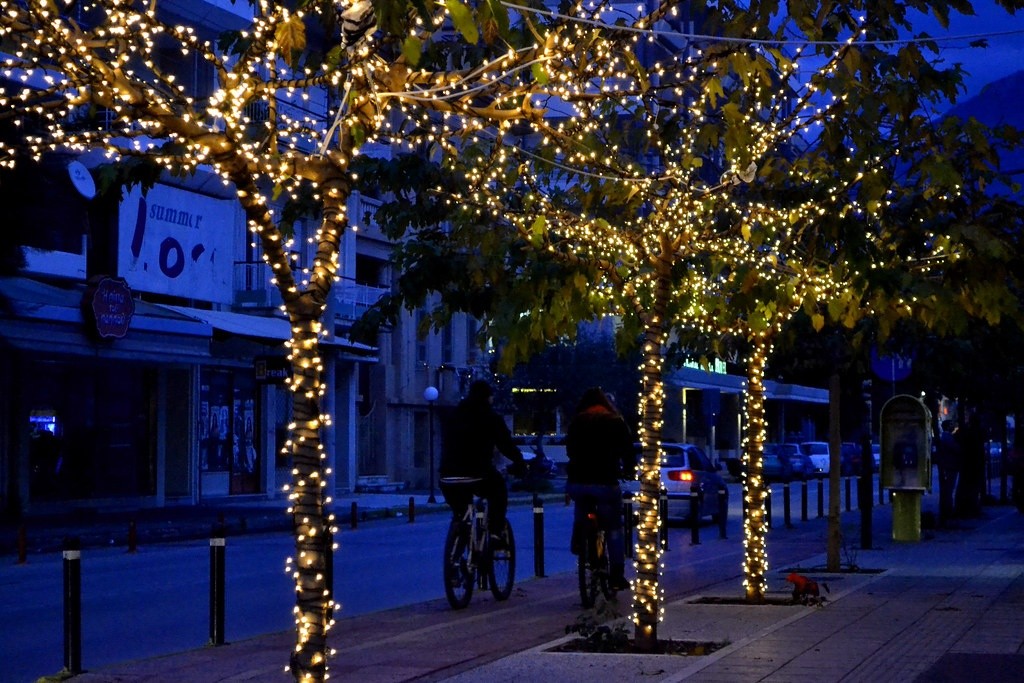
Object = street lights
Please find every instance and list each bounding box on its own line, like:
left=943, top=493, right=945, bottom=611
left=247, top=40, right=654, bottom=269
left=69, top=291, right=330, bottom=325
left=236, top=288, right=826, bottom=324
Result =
left=422, top=384, right=439, bottom=503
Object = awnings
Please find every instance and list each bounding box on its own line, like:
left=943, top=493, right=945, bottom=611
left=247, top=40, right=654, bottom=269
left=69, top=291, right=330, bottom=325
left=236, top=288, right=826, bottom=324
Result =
left=1, top=277, right=215, bottom=371
left=155, top=303, right=380, bottom=357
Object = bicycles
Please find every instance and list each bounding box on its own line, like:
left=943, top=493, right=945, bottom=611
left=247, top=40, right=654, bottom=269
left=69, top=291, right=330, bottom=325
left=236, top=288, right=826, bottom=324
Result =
left=563, top=469, right=636, bottom=602
left=440, top=465, right=531, bottom=608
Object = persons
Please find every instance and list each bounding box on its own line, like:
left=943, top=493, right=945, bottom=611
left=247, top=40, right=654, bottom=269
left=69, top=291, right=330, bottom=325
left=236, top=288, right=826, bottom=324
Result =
left=244, top=417, right=254, bottom=473
left=565, top=386, right=638, bottom=590
left=438, top=380, right=527, bottom=590
left=938, top=411, right=990, bottom=520
left=210, top=412, right=220, bottom=439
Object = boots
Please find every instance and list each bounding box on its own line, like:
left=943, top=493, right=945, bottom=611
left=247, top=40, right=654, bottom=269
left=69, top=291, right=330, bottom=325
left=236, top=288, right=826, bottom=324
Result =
left=608, top=564, right=630, bottom=590
left=571, top=521, right=585, bottom=554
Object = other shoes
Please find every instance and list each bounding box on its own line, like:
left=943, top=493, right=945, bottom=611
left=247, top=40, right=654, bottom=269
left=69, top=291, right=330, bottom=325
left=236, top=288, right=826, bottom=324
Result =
left=489, top=537, right=512, bottom=553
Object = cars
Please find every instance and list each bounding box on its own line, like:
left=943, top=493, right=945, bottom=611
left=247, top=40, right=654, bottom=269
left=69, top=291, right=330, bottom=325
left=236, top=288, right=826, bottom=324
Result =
left=738, top=440, right=881, bottom=480
left=620, top=442, right=730, bottom=525
